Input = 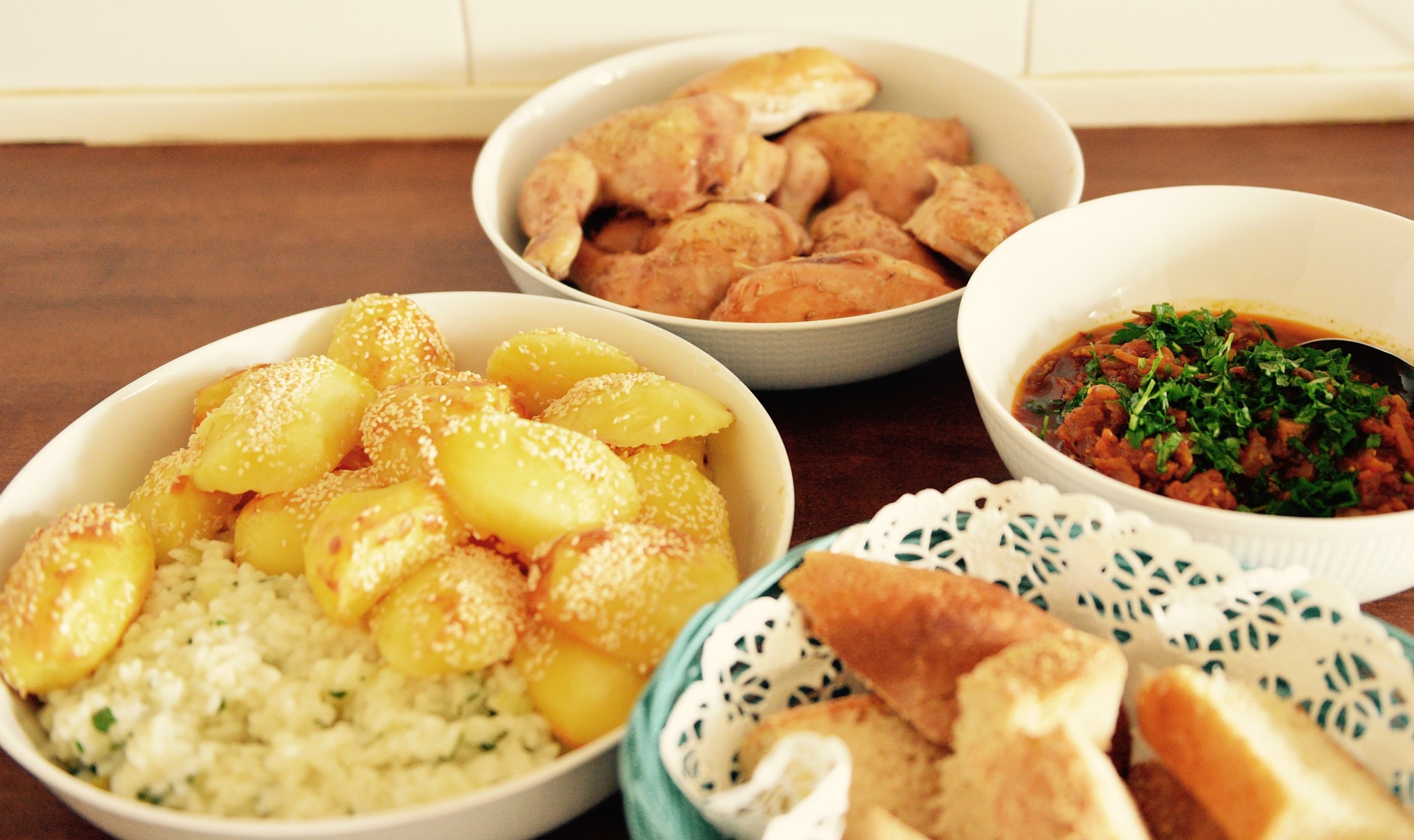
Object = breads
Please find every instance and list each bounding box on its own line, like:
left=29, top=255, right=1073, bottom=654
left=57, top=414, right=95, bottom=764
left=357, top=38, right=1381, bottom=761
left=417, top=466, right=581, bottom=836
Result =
left=736, top=549, right=1413, bottom=839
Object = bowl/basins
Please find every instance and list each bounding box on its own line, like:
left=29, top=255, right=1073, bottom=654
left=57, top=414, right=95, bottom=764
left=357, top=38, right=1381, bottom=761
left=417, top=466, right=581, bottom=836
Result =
left=1, top=292, right=793, bottom=840
left=956, top=188, right=1413, bottom=606
left=470, top=28, right=1085, bottom=387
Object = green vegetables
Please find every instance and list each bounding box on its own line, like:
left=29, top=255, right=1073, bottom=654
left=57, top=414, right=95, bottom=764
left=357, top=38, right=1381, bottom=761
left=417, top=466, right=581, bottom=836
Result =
left=1022, top=302, right=1390, bottom=519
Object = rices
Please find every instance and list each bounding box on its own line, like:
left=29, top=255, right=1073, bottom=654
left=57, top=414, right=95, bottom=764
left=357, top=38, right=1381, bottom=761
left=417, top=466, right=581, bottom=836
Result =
left=30, top=541, right=563, bottom=818
